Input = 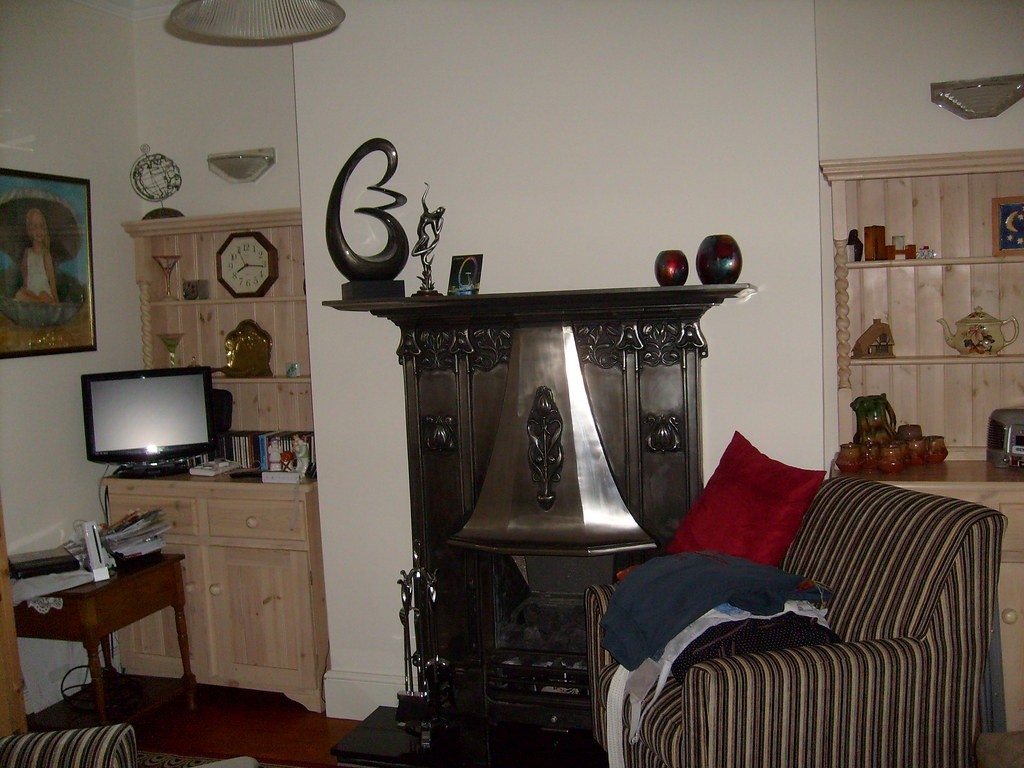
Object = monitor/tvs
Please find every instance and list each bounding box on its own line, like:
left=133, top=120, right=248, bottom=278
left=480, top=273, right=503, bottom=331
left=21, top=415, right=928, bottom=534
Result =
left=81, top=366, right=218, bottom=479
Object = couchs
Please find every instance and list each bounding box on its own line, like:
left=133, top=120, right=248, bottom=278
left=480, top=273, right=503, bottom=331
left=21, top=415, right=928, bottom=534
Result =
left=0, top=722, right=138, bottom=768
left=582, top=474, right=1009, bottom=768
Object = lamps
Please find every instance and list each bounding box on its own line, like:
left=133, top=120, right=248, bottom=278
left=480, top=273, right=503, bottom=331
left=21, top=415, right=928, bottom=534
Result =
left=170, top=0, right=345, bottom=44
left=930, top=72, right=1024, bottom=121
left=207, top=146, right=275, bottom=186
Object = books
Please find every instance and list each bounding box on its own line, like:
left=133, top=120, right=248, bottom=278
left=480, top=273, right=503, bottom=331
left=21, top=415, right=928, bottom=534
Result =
left=215, top=433, right=315, bottom=472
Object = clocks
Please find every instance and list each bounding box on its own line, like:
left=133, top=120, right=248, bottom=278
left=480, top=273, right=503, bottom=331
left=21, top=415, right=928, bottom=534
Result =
left=215, top=231, right=279, bottom=299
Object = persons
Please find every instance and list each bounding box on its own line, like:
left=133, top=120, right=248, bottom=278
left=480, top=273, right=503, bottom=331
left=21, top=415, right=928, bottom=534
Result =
left=268, top=438, right=283, bottom=463
left=294, top=433, right=309, bottom=478
left=411, top=183, right=446, bottom=276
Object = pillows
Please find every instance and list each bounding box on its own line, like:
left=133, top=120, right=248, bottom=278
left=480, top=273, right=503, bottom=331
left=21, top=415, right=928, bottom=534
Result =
left=666, top=430, right=828, bottom=566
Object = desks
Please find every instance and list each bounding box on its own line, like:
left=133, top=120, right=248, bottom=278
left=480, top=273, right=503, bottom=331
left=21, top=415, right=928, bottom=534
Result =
left=13, top=552, right=198, bottom=727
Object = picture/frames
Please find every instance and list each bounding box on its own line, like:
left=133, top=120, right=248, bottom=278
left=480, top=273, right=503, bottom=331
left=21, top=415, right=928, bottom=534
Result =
left=0, top=168, right=98, bottom=360
left=992, top=196, right=1024, bottom=257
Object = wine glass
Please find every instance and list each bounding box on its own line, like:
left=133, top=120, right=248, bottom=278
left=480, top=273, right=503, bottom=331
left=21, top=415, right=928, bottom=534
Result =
left=153, top=255, right=183, bottom=298
left=158, top=331, right=185, bottom=368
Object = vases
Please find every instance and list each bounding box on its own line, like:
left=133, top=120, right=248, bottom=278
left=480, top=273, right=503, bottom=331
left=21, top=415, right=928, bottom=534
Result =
left=655, top=250, right=690, bottom=287
left=696, top=235, right=742, bottom=284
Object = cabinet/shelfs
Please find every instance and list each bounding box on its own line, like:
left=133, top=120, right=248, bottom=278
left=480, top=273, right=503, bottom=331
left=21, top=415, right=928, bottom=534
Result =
left=95, top=207, right=331, bottom=714
left=818, top=148, right=1024, bottom=736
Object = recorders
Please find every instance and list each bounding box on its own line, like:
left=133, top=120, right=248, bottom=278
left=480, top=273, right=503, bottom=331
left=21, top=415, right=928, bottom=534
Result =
left=985, top=408, right=1024, bottom=470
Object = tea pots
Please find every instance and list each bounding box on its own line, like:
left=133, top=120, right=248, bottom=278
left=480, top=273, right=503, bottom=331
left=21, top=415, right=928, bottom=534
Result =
left=850, top=392, right=897, bottom=447
left=937, top=306, right=1019, bottom=358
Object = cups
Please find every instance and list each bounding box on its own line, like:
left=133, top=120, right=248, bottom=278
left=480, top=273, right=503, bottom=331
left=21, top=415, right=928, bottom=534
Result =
left=835, top=424, right=948, bottom=473
